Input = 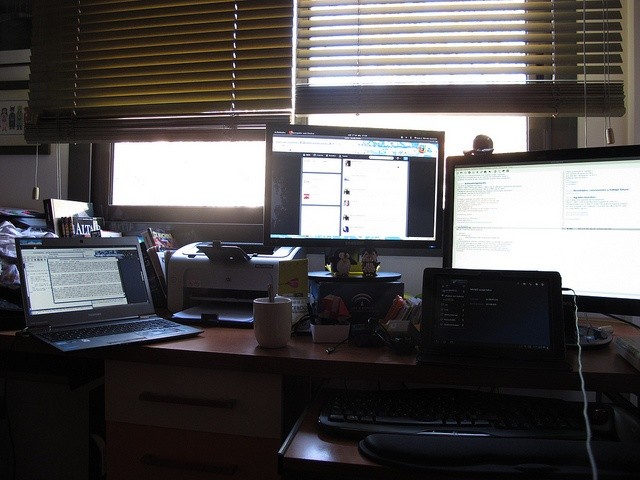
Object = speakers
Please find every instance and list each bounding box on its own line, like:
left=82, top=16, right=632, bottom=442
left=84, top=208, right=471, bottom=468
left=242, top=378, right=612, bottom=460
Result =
left=309, top=283, right=405, bottom=323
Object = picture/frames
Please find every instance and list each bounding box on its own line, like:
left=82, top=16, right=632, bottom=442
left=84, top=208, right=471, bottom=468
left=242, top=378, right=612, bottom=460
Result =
left=1, top=79, right=51, bottom=156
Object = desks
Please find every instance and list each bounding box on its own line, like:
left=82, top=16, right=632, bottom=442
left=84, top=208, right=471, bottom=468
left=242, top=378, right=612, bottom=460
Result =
left=1, top=312, right=639, bottom=480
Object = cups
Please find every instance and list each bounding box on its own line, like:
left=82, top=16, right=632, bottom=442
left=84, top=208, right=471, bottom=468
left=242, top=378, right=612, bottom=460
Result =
left=254, top=298, right=292, bottom=349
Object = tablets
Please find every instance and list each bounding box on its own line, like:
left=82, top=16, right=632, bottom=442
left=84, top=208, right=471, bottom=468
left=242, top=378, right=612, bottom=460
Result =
left=416, top=268, right=566, bottom=370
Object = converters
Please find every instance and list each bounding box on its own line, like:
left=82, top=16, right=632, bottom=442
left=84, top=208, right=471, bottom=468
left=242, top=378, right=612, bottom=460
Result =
left=349, top=316, right=418, bottom=354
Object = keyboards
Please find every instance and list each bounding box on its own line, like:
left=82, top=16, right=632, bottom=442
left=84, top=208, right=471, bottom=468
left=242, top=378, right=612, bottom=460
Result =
left=318, top=388, right=559, bottom=438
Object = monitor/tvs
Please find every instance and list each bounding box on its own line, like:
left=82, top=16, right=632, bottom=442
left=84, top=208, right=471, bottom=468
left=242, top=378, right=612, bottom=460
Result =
left=263, top=124, right=444, bottom=282
left=442, top=143, right=636, bottom=351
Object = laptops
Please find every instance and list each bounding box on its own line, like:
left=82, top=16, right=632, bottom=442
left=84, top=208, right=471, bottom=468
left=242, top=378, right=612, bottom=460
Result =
left=15, top=236, right=204, bottom=353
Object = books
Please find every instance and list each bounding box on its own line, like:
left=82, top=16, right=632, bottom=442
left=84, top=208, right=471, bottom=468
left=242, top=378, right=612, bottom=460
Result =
left=42, top=197, right=104, bottom=240
left=139, top=225, right=176, bottom=314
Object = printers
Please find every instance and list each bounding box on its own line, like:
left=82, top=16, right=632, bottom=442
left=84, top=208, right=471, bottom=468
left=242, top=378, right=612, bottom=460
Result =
left=165, top=239, right=307, bottom=330
